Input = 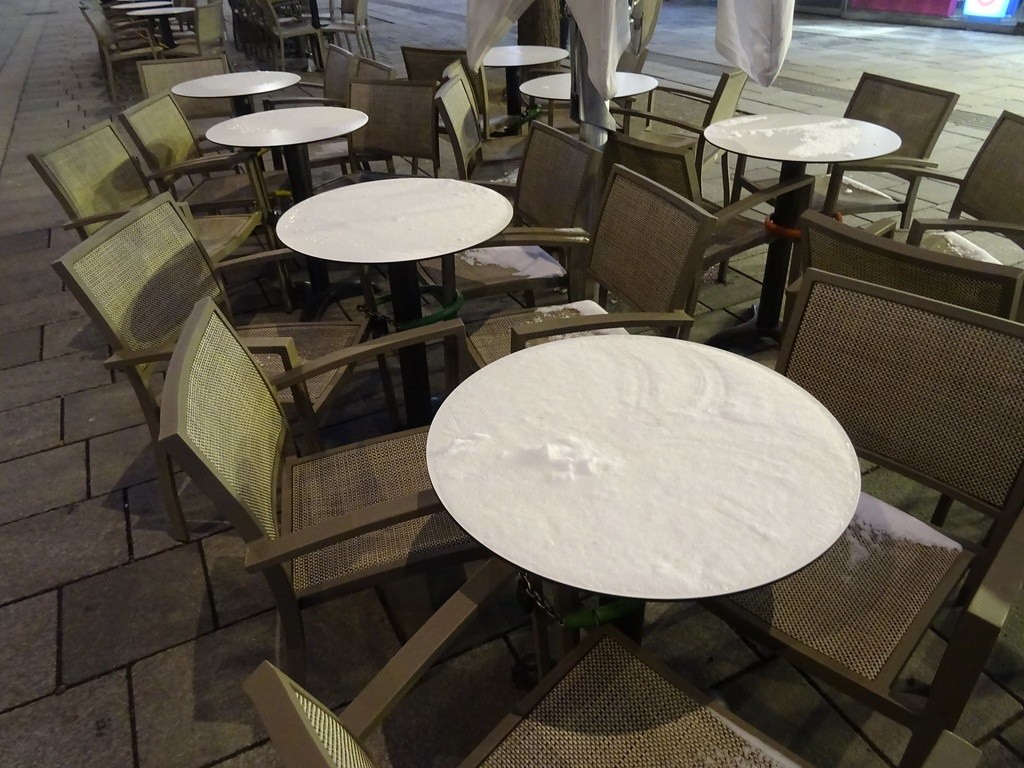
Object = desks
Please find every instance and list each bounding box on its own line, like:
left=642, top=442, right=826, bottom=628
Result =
left=110, top=1, right=173, bottom=36
left=126, top=7, right=196, bottom=59
left=426, top=334, right=862, bottom=646
left=276, top=177, right=516, bottom=429
left=171, top=71, right=301, bottom=172
left=481, top=45, right=569, bottom=138
left=519, top=72, right=659, bottom=101
left=206, top=106, right=382, bottom=322
left=703, top=112, right=902, bottom=343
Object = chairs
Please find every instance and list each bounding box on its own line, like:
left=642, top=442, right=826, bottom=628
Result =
left=25, top=0, right=1024, bottom=768
